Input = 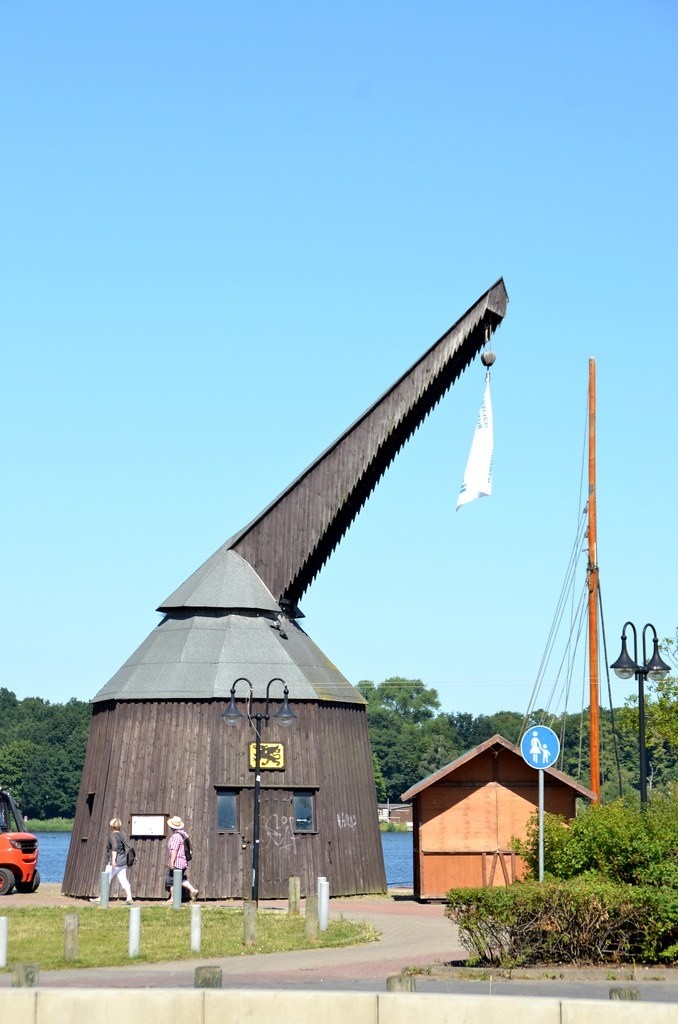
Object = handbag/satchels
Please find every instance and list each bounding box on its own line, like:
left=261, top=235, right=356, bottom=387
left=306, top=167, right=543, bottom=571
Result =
left=126, top=848, right=136, bottom=865
left=174, top=832, right=192, bottom=861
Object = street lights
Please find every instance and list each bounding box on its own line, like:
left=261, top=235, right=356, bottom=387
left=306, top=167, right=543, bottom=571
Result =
left=611, top=622, right=672, bottom=812
left=220, top=677, right=298, bottom=908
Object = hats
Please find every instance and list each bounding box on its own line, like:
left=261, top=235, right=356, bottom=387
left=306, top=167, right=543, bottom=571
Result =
left=167, top=816, right=184, bottom=829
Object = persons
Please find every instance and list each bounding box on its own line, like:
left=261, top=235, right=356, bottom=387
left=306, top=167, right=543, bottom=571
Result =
left=89, top=818, right=133, bottom=905
left=162, top=815, right=198, bottom=906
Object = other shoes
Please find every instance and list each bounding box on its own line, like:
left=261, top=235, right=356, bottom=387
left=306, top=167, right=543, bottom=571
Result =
left=189, top=889, right=199, bottom=902
left=122, top=900, right=134, bottom=905
left=167, top=898, right=173, bottom=902
left=89, top=898, right=100, bottom=905
